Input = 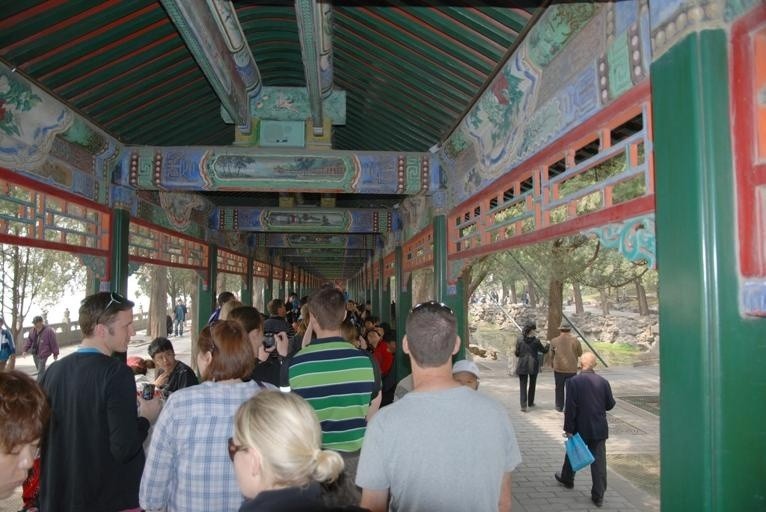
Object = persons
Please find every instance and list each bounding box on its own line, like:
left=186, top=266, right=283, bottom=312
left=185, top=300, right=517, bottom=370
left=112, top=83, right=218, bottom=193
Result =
left=126, top=283, right=522, bottom=512
left=21, top=291, right=164, bottom=512
left=514, top=321, right=551, bottom=413
left=0, top=369, right=51, bottom=512
left=554, top=351, right=617, bottom=507
left=549, top=321, right=583, bottom=412
left=0, top=318, right=16, bottom=369
left=22, top=315, right=59, bottom=383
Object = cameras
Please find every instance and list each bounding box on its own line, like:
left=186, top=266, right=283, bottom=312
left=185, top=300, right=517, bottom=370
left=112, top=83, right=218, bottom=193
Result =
left=262, top=333, right=275, bottom=348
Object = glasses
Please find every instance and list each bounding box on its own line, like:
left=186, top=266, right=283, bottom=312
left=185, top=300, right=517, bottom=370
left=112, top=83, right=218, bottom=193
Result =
left=228, top=438, right=248, bottom=462
left=96, top=291, right=124, bottom=324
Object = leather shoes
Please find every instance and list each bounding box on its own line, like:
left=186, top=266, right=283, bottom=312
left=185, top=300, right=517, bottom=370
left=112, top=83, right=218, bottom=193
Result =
left=555, top=473, right=574, bottom=489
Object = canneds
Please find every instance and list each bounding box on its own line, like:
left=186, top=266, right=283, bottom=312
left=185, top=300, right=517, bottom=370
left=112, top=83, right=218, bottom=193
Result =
left=143, top=383, right=155, bottom=400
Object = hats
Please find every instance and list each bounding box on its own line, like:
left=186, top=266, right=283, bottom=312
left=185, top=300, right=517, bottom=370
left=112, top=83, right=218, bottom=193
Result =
left=32, top=316, right=42, bottom=323
left=558, top=321, right=572, bottom=329
left=452, top=360, right=480, bottom=380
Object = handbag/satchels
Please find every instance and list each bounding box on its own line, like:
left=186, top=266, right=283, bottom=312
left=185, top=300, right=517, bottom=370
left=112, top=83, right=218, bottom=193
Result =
left=563, top=433, right=595, bottom=471
left=30, top=343, right=37, bottom=354
